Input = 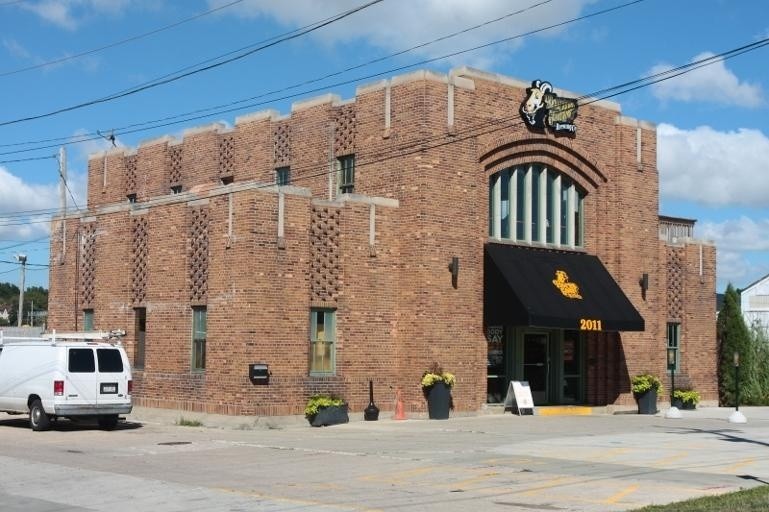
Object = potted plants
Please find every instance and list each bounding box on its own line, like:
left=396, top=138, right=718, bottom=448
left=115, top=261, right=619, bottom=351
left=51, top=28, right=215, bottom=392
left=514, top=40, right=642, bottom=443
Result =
left=420, top=363, right=456, bottom=419
left=631, top=370, right=664, bottom=414
left=670, top=388, right=701, bottom=410
left=305, top=392, right=350, bottom=427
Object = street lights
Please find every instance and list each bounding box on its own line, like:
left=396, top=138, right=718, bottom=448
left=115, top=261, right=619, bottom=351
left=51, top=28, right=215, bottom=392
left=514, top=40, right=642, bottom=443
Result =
left=733, top=352, right=738, bottom=411
left=17, top=255, right=26, bottom=327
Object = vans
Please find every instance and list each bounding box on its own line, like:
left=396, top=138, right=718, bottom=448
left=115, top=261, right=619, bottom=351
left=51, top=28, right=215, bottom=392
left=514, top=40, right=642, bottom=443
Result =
left=0, top=340, right=132, bottom=431
